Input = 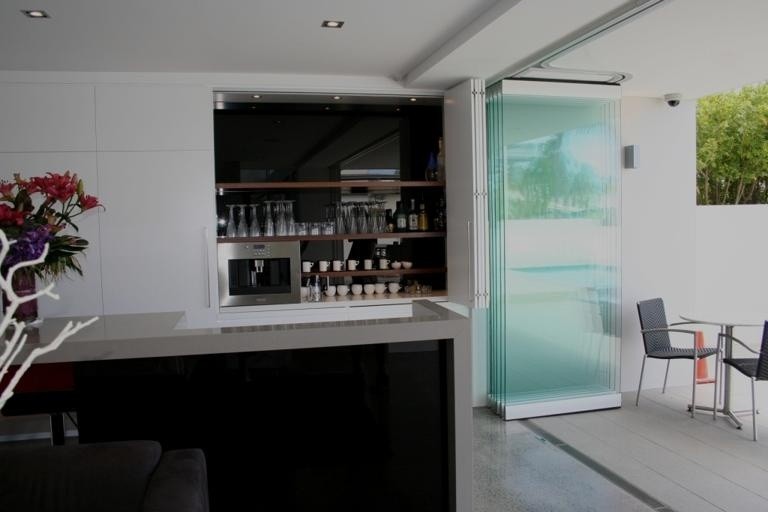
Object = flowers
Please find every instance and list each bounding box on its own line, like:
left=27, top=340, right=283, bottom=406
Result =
left=0, top=170, right=106, bottom=287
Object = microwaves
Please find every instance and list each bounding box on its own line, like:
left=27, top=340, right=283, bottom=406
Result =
left=217, top=239, right=302, bottom=307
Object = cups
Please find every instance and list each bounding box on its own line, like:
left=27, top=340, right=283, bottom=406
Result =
left=296, top=221, right=335, bottom=235
left=300, top=259, right=410, bottom=301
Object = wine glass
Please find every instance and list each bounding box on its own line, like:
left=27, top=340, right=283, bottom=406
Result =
left=226, top=200, right=295, bottom=238
left=324, top=201, right=390, bottom=234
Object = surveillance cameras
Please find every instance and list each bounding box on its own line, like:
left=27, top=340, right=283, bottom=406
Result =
left=666, top=93, right=682, bottom=106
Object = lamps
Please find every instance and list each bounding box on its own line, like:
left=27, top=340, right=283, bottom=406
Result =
left=664, top=93, right=680, bottom=107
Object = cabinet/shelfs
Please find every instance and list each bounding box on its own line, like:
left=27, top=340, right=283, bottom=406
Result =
left=205, top=91, right=448, bottom=314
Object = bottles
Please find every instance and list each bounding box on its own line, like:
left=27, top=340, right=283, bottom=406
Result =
left=425, top=136, right=445, bottom=181
left=386, top=198, right=444, bottom=233
left=375, top=249, right=387, bottom=269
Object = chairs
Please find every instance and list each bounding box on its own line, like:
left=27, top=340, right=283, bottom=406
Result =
left=636, top=298, right=723, bottom=418
left=0, top=440, right=210, bottom=512
left=713, top=320, right=768, bottom=441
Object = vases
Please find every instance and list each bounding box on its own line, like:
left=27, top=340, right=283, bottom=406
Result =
left=2, top=270, right=37, bottom=326
